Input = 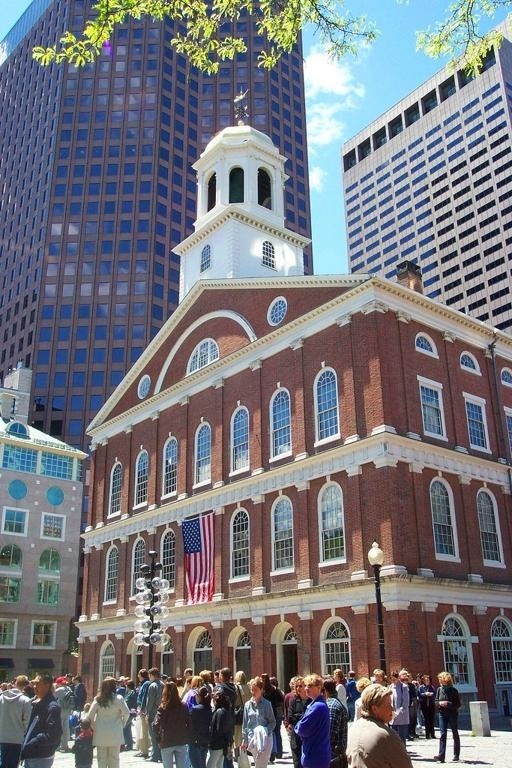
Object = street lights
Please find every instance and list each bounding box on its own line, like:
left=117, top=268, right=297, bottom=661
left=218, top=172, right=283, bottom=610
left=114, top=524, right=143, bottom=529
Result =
left=134, top=551, right=172, bottom=672
left=366, top=542, right=388, bottom=674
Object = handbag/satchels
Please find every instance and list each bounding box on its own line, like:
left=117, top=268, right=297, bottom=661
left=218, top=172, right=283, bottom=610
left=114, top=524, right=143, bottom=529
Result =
left=234, top=706, right=244, bottom=725
left=331, top=753, right=348, bottom=768
left=238, top=749, right=251, bottom=768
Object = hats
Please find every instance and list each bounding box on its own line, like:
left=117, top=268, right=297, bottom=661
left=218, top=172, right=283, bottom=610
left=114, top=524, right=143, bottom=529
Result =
left=81, top=718, right=91, bottom=728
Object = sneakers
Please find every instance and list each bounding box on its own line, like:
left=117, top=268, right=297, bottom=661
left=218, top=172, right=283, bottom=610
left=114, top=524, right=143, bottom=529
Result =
left=434, top=755, right=445, bottom=762
left=270, top=752, right=276, bottom=762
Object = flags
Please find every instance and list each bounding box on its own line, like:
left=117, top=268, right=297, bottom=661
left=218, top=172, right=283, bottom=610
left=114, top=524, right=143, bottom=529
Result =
left=180, top=512, right=217, bottom=607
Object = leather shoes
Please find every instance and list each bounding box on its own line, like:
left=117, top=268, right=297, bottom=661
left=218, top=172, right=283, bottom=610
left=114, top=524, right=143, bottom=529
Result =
left=134, top=752, right=148, bottom=757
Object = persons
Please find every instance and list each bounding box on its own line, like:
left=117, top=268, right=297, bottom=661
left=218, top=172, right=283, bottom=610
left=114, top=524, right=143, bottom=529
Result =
left=434, top=671, right=462, bottom=763
left=1, top=664, right=439, bottom=767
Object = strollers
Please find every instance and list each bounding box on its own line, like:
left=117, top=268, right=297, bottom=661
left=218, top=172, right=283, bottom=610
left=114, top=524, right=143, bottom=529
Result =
left=66, top=703, right=81, bottom=740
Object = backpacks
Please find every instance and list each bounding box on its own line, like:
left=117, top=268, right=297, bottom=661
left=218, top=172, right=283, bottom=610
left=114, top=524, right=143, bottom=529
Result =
left=60, top=686, right=76, bottom=713
